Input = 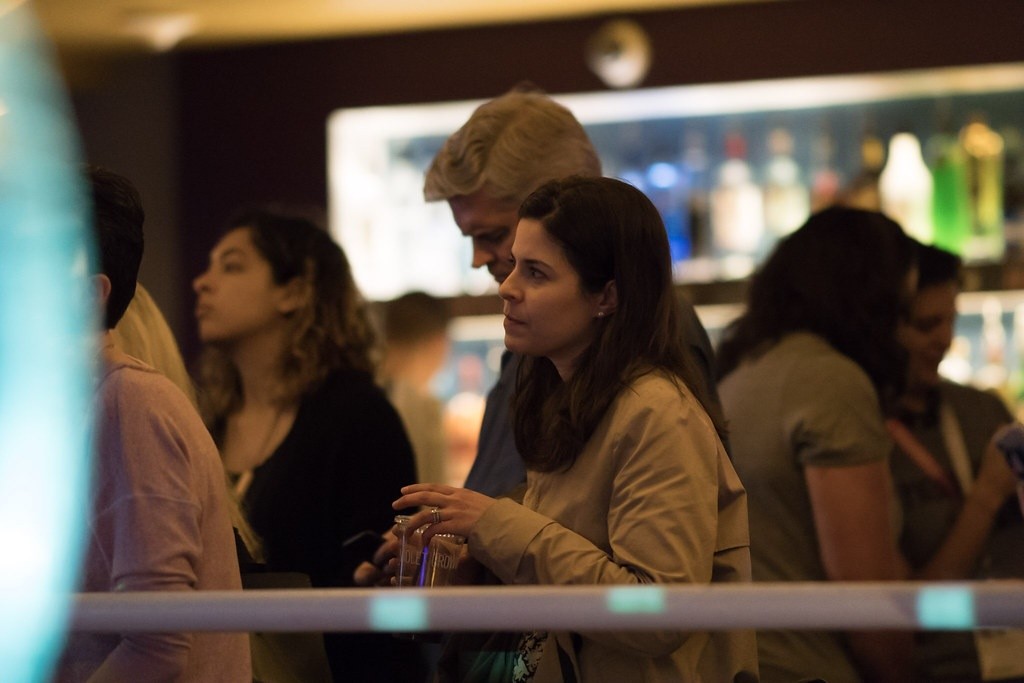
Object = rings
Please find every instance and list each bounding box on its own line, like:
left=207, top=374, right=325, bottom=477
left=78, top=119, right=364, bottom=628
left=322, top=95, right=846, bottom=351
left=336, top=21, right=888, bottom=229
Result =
left=431, top=506, right=441, bottom=524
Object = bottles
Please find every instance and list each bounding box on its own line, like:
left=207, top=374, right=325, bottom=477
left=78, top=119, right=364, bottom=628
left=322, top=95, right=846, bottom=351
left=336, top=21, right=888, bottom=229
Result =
left=612, top=104, right=1006, bottom=269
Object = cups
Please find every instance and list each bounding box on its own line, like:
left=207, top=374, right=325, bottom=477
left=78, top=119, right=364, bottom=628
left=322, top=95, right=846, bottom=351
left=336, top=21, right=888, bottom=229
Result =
left=393, top=515, right=466, bottom=645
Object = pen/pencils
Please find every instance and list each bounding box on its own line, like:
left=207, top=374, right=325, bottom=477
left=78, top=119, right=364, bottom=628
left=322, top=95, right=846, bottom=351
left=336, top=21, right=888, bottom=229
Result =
left=942, top=405, right=973, bottom=497
left=882, top=417, right=959, bottom=498
left=410, top=546, right=429, bottom=639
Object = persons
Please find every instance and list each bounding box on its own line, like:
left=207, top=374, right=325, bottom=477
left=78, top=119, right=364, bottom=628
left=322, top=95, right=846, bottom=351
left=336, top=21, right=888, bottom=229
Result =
left=53, top=85, right=1023, bottom=683
